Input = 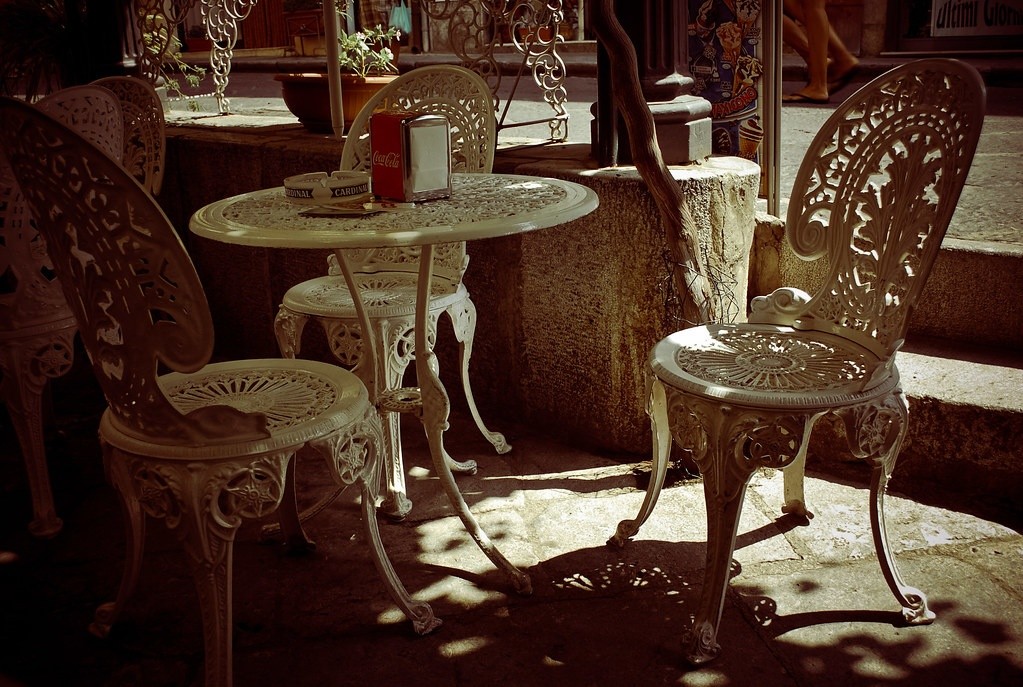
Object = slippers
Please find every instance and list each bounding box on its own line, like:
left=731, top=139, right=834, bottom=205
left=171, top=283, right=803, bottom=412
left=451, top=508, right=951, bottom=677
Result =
left=827, top=64, right=861, bottom=96
left=782, top=93, right=830, bottom=104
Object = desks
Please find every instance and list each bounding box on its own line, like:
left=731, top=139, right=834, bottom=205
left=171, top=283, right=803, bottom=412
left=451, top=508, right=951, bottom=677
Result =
left=189, top=173, right=601, bottom=514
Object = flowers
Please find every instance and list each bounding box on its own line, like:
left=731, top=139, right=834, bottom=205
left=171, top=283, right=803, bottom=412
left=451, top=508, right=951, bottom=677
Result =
left=336, top=23, right=402, bottom=77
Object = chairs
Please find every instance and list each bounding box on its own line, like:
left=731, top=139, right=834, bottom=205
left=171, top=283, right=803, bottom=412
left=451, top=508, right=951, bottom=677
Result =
left=0, top=95, right=442, bottom=687
left=271, top=63, right=513, bottom=516
left=89, top=76, right=166, bottom=205
left=607, top=57, right=987, bottom=664
left=2, top=84, right=127, bottom=537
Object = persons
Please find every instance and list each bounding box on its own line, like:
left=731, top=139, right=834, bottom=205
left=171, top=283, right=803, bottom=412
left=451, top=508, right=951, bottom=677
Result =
left=782, top=0, right=860, bottom=104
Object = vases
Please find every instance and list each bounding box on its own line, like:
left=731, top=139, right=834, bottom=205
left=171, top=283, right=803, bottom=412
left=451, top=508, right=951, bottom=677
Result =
left=274, top=72, right=402, bottom=134
